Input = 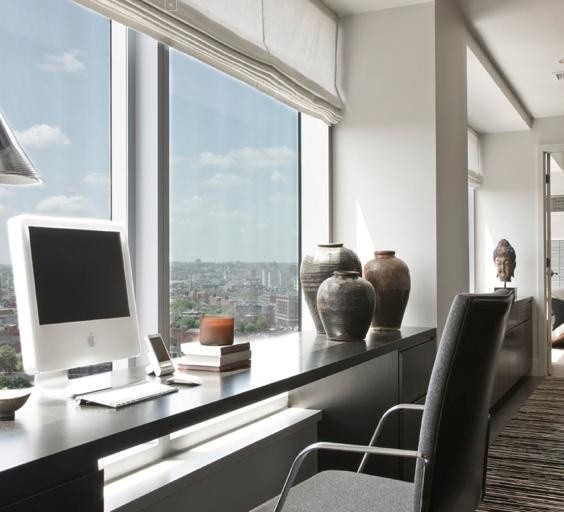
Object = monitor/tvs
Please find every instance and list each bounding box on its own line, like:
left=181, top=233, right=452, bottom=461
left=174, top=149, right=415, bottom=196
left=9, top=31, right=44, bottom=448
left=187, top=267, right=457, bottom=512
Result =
left=7, top=214, right=141, bottom=398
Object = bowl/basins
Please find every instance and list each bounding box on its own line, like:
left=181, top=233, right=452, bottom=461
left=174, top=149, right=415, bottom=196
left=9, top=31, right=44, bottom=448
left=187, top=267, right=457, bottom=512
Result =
left=0, top=388, right=31, bottom=417
left=198, top=316, right=234, bottom=346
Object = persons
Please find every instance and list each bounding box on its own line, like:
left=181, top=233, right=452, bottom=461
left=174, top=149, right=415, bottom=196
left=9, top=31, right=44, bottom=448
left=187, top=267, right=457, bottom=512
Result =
left=493, top=239, right=516, bottom=281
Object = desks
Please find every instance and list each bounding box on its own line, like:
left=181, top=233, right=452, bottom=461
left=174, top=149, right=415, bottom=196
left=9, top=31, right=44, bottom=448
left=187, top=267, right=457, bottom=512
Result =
left=0, top=325, right=438, bottom=512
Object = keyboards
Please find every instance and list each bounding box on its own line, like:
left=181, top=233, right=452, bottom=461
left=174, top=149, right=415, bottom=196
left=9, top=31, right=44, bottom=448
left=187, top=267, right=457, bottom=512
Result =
left=74, top=382, right=179, bottom=409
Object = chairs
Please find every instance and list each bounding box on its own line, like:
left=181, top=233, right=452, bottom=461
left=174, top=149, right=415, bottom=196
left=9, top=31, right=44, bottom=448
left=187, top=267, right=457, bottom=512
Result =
left=242, top=286, right=517, bottom=510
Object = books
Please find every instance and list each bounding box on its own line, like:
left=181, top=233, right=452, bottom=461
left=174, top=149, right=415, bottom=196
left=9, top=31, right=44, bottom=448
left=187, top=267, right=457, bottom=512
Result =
left=177, top=341, right=251, bottom=372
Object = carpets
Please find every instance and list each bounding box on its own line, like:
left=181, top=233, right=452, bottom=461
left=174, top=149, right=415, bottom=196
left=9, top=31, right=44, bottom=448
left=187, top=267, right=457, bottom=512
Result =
left=475, top=375, right=564, bottom=511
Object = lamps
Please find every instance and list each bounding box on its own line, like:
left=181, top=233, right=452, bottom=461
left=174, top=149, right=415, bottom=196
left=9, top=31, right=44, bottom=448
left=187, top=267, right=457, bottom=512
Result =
left=0, top=116, right=44, bottom=187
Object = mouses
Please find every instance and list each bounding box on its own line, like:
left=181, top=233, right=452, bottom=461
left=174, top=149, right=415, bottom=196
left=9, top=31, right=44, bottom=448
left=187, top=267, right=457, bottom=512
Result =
left=167, top=375, right=201, bottom=385
